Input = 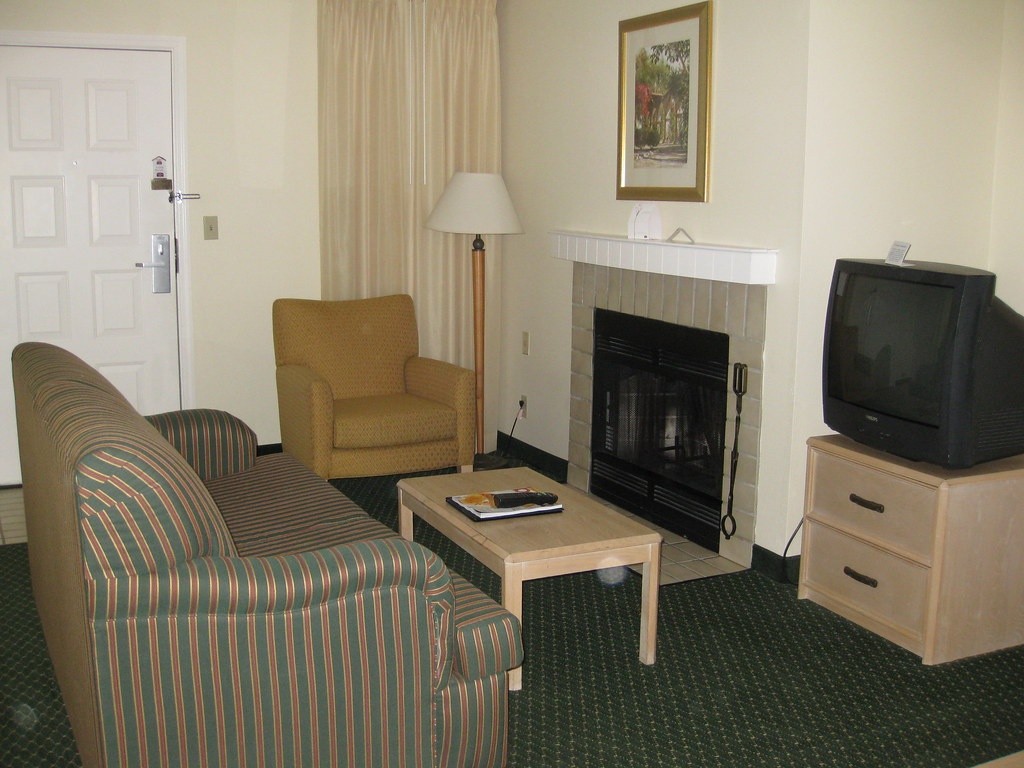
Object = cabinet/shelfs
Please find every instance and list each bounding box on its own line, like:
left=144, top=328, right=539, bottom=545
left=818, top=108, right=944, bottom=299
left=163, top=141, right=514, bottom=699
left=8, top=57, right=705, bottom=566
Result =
left=797, top=434, right=1024, bottom=667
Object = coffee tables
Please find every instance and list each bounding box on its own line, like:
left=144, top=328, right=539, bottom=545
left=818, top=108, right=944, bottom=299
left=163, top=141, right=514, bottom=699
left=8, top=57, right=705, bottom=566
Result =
left=396, top=465, right=663, bottom=694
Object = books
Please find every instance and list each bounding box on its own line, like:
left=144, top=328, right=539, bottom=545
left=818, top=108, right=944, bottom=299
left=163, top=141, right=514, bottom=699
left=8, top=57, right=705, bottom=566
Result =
left=452, top=486, right=562, bottom=519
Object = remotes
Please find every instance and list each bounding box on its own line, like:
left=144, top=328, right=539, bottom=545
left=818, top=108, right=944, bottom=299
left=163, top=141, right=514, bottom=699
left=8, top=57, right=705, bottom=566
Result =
left=494, top=491, right=559, bottom=508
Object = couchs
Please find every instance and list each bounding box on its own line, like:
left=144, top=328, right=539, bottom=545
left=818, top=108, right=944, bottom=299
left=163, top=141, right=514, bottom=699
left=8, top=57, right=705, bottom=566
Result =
left=270, top=292, right=480, bottom=480
left=10, top=341, right=527, bottom=768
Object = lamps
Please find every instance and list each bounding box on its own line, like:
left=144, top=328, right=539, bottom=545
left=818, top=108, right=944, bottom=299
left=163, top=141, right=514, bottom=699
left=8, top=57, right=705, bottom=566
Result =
left=429, top=172, right=526, bottom=472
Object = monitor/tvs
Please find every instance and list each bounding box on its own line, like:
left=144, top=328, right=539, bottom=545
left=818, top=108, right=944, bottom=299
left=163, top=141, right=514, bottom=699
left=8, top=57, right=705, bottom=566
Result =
left=823, top=259, right=1024, bottom=470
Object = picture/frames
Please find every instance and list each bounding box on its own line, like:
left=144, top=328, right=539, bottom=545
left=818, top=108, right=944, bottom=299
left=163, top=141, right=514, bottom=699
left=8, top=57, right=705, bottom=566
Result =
left=616, top=0, right=713, bottom=206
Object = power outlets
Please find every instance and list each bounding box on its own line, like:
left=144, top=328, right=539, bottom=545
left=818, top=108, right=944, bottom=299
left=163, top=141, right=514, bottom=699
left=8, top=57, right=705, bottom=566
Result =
left=521, top=329, right=531, bottom=356
left=521, top=395, right=528, bottom=418
